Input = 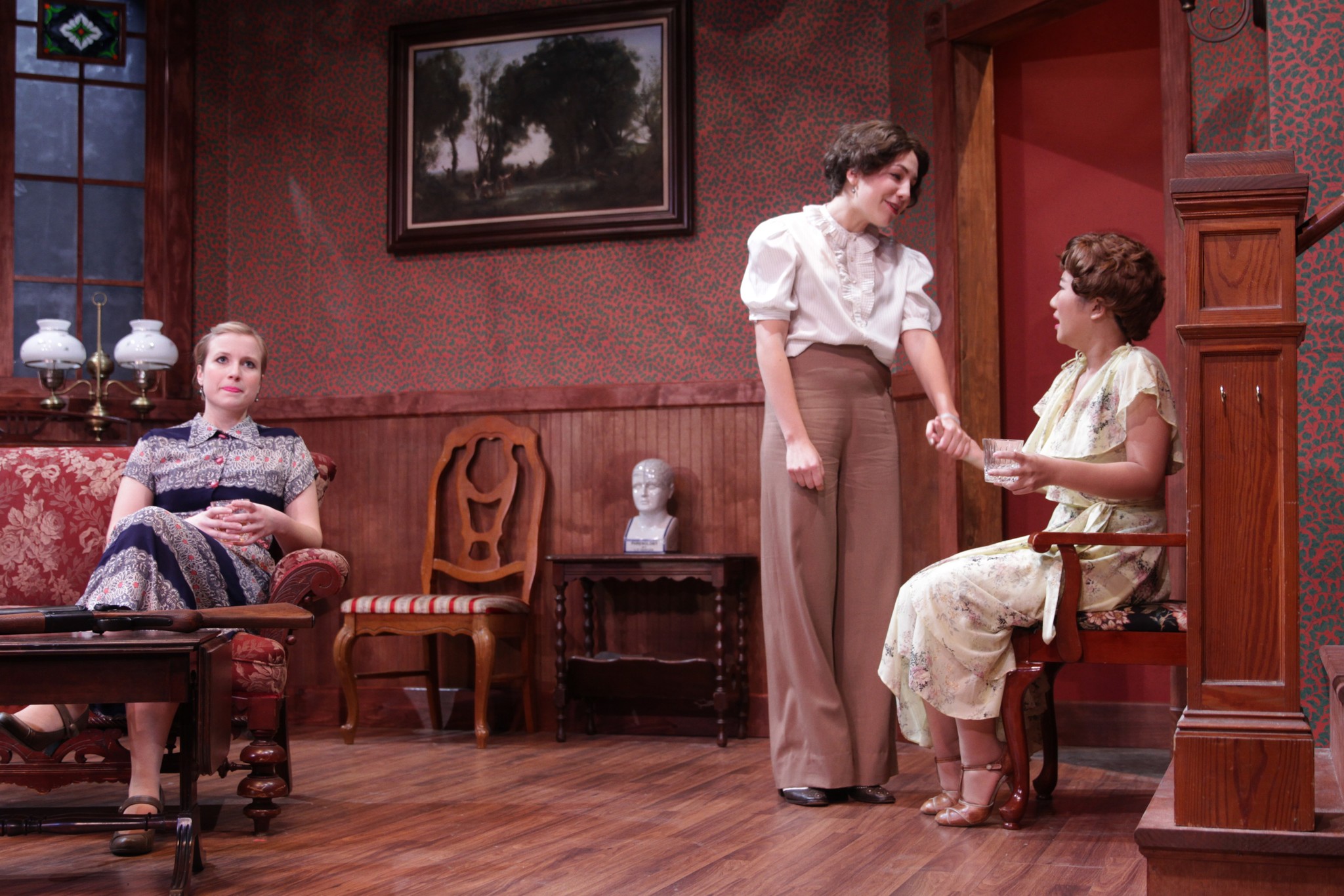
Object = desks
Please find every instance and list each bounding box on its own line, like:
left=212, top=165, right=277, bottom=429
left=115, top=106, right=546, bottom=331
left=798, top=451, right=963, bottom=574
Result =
left=546, top=552, right=759, bottom=747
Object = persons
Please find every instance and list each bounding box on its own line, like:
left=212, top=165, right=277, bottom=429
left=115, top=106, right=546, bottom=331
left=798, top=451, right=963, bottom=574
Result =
left=0, top=322, right=322, bottom=858
left=622, top=458, right=680, bottom=555
left=740, top=121, right=968, bottom=806
left=877, top=231, right=1187, bottom=826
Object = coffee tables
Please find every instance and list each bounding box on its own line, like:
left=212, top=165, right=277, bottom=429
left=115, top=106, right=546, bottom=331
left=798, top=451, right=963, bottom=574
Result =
left=0, top=628, right=243, bottom=896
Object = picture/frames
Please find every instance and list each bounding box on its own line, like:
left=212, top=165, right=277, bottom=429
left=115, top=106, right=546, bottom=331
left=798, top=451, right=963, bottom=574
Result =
left=385, top=0, right=689, bottom=251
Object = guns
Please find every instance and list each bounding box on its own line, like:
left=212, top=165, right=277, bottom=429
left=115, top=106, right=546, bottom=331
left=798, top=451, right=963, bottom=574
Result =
left=0, top=601, right=315, bottom=636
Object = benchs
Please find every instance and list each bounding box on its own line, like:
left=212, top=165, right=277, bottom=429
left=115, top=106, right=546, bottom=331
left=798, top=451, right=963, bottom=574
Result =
left=0, top=448, right=344, bottom=842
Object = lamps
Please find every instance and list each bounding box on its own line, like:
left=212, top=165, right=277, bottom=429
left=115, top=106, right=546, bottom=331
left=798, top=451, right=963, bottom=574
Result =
left=22, top=291, right=179, bottom=443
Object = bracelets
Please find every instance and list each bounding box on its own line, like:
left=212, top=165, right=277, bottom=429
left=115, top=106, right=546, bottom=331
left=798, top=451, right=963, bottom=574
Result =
left=941, top=411, right=962, bottom=425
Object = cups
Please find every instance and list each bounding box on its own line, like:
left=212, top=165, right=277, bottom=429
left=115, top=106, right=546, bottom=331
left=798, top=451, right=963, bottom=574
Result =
left=210, top=500, right=252, bottom=543
left=982, top=439, right=1026, bottom=485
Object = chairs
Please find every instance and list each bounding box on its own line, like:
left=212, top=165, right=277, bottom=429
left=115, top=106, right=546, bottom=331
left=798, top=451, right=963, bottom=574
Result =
left=333, top=414, right=548, bottom=748
left=998, top=531, right=1188, bottom=830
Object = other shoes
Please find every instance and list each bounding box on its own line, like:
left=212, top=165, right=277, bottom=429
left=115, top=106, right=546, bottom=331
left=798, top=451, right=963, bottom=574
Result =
left=847, top=784, right=897, bottom=804
left=778, top=787, right=828, bottom=807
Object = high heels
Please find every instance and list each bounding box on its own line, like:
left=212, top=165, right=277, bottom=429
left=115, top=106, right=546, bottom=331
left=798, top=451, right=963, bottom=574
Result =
left=110, top=785, right=165, bottom=855
left=920, top=757, right=964, bottom=815
left=935, top=744, right=1014, bottom=827
left=0, top=703, right=93, bottom=756
